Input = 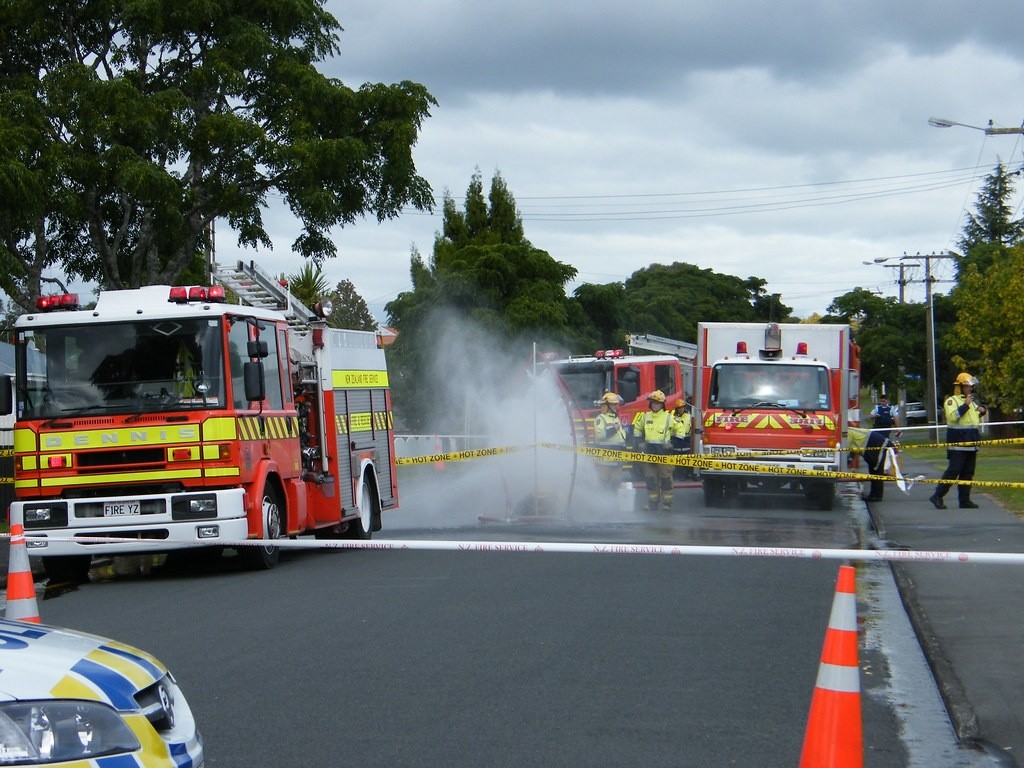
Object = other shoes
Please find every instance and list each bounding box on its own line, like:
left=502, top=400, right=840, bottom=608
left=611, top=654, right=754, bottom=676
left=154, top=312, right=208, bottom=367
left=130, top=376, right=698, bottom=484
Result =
left=960, top=500, right=978, bottom=508
left=929, top=495, right=946, bottom=508
left=663, top=502, right=670, bottom=510
left=862, top=495, right=872, bottom=501
left=873, top=497, right=881, bottom=502
left=644, top=501, right=659, bottom=510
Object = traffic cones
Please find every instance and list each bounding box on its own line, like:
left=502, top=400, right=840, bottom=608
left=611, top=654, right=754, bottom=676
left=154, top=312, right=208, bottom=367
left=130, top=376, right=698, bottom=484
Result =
left=5, top=523, right=40, bottom=625
left=433, top=437, right=445, bottom=471
left=798, top=565, right=864, bottom=768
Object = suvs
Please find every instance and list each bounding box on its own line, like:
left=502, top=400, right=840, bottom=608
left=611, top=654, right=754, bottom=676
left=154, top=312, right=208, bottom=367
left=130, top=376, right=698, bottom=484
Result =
left=892, top=403, right=944, bottom=426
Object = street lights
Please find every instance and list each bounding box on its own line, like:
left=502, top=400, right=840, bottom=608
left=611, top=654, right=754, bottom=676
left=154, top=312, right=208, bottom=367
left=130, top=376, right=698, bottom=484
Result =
left=873, top=251, right=960, bottom=449
left=862, top=261, right=920, bottom=403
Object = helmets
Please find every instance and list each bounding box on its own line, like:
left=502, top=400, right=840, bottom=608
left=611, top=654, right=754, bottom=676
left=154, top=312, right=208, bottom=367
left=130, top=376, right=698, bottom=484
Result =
left=953, top=372, right=974, bottom=385
left=601, top=392, right=621, bottom=404
left=675, top=399, right=685, bottom=408
left=648, top=390, right=665, bottom=402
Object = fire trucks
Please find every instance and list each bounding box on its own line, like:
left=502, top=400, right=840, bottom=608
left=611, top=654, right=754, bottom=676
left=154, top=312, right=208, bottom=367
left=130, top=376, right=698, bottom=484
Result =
left=535, top=321, right=860, bottom=511
left=6, top=260, right=400, bottom=583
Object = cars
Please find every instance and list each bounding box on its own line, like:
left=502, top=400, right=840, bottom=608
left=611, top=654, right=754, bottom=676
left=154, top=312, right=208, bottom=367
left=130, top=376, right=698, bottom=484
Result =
left=0, top=618, right=204, bottom=768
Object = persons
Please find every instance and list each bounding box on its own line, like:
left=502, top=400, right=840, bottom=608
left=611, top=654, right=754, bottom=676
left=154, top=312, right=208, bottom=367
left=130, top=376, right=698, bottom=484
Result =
left=847, top=428, right=892, bottom=501
left=670, top=400, right=693, bottom=480
left=870, top=396, right=899, bottom=439
left=930, top=373, right=986, bottom=508
left=594, top=393, right=626, bottom=472
left=633, top=391, right=685, bottom=511
